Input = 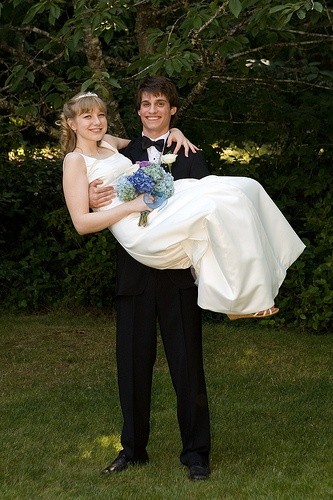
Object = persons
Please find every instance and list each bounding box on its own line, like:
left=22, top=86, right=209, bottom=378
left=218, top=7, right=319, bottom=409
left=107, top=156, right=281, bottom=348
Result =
left=87, top=75, right=212, bottom=481
left=56, top=91, right=307, bottom=321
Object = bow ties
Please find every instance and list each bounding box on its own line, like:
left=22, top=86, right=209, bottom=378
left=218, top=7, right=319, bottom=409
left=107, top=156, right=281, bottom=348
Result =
left=141, top=135, right=164, bottom=153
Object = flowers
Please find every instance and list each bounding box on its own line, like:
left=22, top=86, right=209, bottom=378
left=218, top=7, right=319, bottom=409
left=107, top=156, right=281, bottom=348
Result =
left=115, top=161, right=176, bottom=227
left=160, top=150, right=178, bottom=173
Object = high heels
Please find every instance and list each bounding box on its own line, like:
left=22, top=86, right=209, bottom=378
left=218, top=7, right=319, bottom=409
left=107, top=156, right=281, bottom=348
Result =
left=225, top=304, right=279, bottom=321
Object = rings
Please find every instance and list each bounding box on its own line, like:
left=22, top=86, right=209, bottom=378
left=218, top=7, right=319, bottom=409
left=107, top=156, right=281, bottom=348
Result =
left=94, top=187, right=97, bottom=193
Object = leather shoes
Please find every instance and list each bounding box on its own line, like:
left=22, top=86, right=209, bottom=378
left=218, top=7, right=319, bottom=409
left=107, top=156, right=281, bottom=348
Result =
left=187, top=465, right=211, bottom=482
left=100, top=449, right=140, bottom=476
